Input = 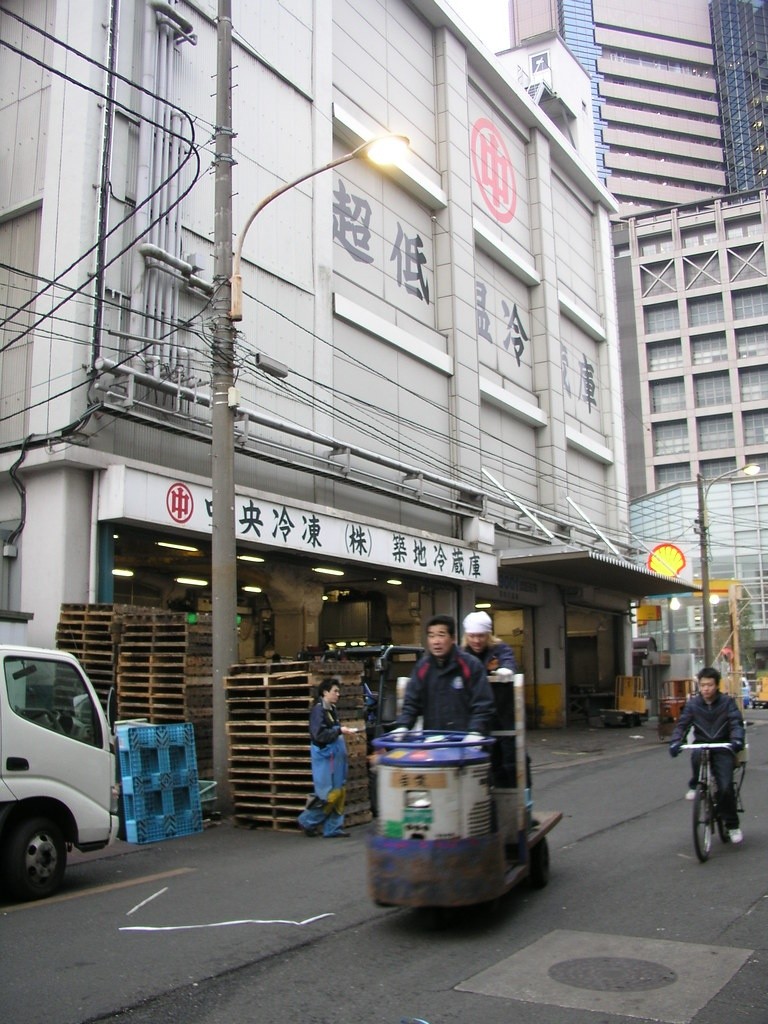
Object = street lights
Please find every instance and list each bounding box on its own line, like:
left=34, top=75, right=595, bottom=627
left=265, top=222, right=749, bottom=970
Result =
left=693, top=460, right=764, bottom=670
left=209, top=132, right=418, bottom=817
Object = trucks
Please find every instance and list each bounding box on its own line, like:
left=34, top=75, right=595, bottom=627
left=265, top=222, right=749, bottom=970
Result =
left=0, top=643, right=123, bottom=903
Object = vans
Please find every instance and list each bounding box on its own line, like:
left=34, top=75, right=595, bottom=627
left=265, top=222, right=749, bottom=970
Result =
left=741, top=677, right=751, bottom=710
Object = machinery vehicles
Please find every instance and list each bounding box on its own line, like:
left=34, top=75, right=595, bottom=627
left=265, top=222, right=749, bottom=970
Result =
left=599, top=675, right=651, bottom=729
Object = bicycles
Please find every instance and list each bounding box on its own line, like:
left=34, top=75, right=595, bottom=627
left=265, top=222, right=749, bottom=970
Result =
left=674, top=741, right=748, bottom=862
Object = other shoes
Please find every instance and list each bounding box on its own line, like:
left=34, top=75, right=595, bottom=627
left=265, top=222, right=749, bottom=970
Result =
left=300, top=823, right=318, bottom=836
left=729, top=829, right=742, bottom=843
left=335, top=831, right=350, bottom=837
left=531, top=819, right=540, bottom=828
left=686, top=790, right=695, bottom=800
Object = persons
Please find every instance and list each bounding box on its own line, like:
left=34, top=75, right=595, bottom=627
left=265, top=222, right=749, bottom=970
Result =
left=297, top=679, right=358, bottom=837
left=669, top=668, right=745, bottom=843
left=391, top=615, right=494, bottom=750
left=461, top=611, right=516, bottom=759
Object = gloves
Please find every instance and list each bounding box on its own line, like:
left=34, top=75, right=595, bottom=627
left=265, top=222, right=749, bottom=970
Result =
left=464, top=732, right=485, bottom=750
left=491, top=667, right=514, bottom=675
left=732, top=739, right=744, bottom=753
left=670, top=742, right=682, bottom=757
left=389, top=727, right=409, bottom=741
